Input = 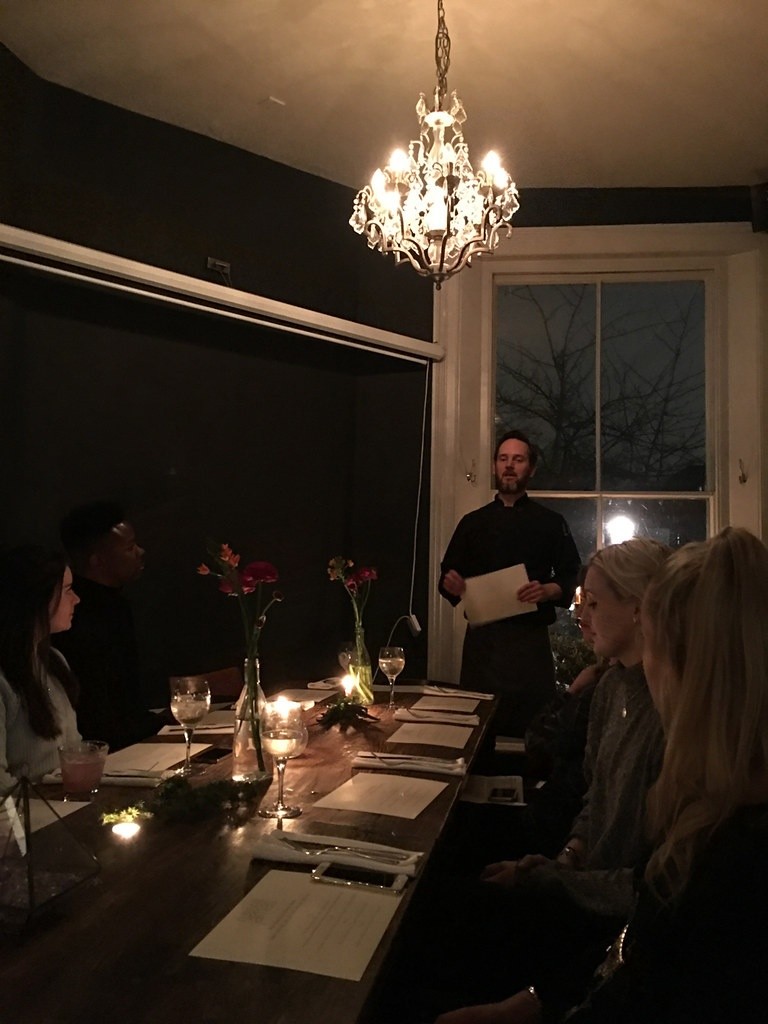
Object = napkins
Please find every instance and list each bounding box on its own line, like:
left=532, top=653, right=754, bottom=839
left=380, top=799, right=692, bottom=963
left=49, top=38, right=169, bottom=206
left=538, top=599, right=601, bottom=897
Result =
left=251, top=830, right=424, bottom=877
left=351, top=751, right=467, bottom=776
left=308, top=677, right=341, bottom=688
left=392, top=708, right=480, bottom=726
left=423, top=685, right=494, bottom=700
left=42, top=768, right=175, bottom=787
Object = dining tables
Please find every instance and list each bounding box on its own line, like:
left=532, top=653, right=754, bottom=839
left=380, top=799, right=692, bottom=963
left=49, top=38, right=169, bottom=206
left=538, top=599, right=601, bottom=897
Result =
left=0, top=676, right=504, bottom=1024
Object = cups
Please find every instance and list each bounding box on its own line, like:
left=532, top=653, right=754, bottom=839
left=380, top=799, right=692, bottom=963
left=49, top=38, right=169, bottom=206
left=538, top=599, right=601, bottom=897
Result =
left=58, top=740, right=109, bottom=800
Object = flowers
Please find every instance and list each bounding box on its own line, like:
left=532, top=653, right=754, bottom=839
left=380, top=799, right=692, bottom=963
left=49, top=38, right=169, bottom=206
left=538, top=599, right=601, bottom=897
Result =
left=196, top=543, right=284, bottom=773
left=327, top=556, right=379, bottom=701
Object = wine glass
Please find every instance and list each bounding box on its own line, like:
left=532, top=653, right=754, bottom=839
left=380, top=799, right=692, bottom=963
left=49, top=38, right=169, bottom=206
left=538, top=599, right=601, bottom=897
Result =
left=258, top=704, right=308, bottom=818
left=378, top=647, right=405, bottom=710
left=170, top=676, right=211, bottom=778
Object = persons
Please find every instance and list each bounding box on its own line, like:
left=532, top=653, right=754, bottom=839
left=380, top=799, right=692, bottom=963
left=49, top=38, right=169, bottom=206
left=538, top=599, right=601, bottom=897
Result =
left=356, top=524, right=768, bottom=1023
left=437, top=431, right=581, bottom=736
left=0, top=544, right=82, bottom=800
left=36, top=501, right=244, bottom=754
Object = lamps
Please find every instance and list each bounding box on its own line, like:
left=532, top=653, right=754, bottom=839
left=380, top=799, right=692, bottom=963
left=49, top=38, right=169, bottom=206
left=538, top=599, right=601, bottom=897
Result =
left=348, top=0, right=519, bottom=291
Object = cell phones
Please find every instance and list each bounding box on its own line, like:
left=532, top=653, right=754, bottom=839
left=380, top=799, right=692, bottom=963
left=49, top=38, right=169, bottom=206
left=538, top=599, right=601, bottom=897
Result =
left=488, top=788, right=518, bottom=802
left=193, top=747, right=234, bottom=763
left=310, top=863, right=411, bottom=894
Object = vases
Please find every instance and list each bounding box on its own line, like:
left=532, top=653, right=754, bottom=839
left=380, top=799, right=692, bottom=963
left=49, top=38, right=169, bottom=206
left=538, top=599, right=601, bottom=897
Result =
left=232, top=660, right=274, bottom=783
left=344, top=633, right=375, bottom=707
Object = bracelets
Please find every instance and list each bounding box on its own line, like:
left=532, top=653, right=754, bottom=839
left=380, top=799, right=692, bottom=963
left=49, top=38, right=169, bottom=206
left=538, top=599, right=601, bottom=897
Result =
left=558, top=846, right=580, bottom=868
left=525, top=984, right=542, bottom=1011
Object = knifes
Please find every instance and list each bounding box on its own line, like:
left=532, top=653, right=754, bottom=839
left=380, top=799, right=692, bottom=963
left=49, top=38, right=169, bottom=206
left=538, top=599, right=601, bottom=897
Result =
left=356, top=755, right=464, bottom=763
left=278, top=837, right=407, bottom=860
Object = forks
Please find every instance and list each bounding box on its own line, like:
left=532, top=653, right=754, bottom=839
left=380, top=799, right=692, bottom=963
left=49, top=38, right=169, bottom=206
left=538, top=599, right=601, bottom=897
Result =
left=371, top=751, right=464, bottom=768
left=282, top=838, right=399, bottom=864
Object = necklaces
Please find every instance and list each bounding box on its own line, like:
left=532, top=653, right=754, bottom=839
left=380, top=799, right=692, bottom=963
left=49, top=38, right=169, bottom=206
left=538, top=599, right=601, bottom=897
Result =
left=46, top=685, right=55, bottom=698
